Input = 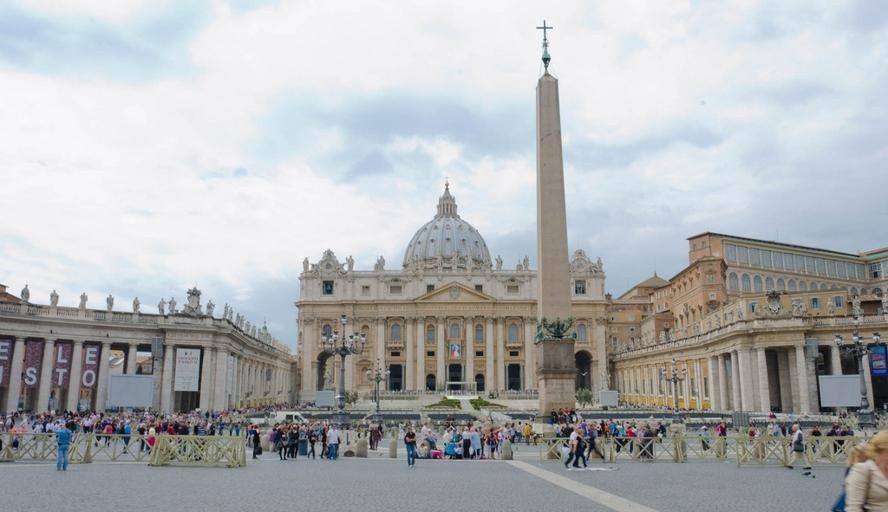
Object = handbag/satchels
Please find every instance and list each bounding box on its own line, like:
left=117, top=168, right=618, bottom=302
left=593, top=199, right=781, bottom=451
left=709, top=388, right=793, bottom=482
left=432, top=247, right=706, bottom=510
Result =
left=833, top=492, right=868, bottom=512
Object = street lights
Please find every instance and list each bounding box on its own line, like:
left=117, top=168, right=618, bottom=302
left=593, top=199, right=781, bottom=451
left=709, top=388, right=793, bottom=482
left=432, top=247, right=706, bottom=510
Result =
left=366, top=357, right=393, bottom=423
left=321, top=313, right=366, bottom=428
left=834, top=316, right=881, bottom=428
left=662, top=355, right=687, bottom=423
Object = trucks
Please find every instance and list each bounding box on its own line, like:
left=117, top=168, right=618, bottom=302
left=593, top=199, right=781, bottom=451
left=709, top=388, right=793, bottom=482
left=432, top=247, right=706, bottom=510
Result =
left=248, top=410, right=309, bottom=427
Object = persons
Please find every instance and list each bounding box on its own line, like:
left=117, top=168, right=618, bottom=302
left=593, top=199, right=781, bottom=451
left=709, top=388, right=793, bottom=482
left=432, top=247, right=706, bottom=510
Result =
left=56, top=423, right=73, bottom=470
left=20, top=284, right=30, bottom=302
left=157, top=296, right=215, bottom=316
left=78, top=292, right=88, bottom=310
left=523, top=255, right=529, bottom=270
left=831, top=441, right=868, bottom=512
left=107, top=295, right=114, bottom=312
left=346, top=256, right=355, bottom=270
left=302, top=256, right=309, bottom=271
left=495, top=254, right=503, bottom=270
left=845, top=429, right=888, bottom=512
left=1, top=407, right=261, bottom=460
left=550, top=406, right=855, bottom=470
left=223, top=303, right=256, bottom=338
left=270, top=420, right=383, bottom=460
left=404, top=421, right=537, bottom=467
left=50, top=289, right=58, bottom=308
left=755, top=294, right=888, bottom=317
left=133, top=297, right=140, bottom=313
left=377, top=256, right=385, bottom=270
